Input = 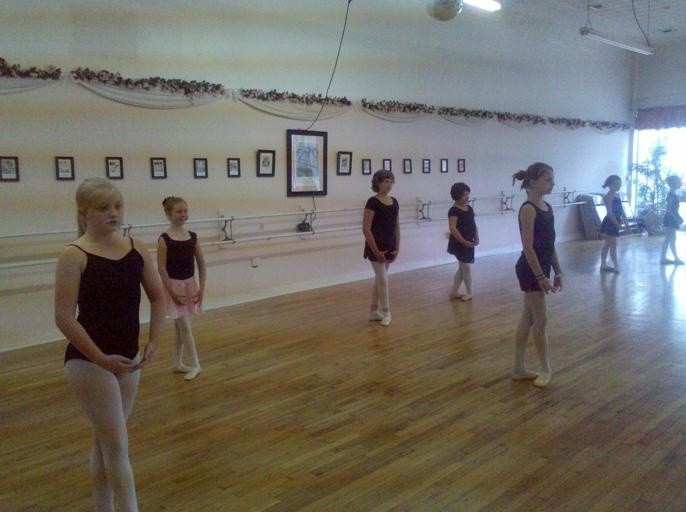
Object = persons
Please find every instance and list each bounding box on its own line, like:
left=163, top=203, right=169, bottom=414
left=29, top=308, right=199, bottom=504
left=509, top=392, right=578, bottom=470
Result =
left=599, top=175, right=633, bottom=273
left=511, top=163, right=564, bottom=387
left=446, top=182, right=480, bottom=301
left=157, top=196, right=207, bottom=381
left=361, top=169, right=400, bottom=327
left=54, top=177, right=168, bottom=511
left=660, top=176, right=685, bottom=265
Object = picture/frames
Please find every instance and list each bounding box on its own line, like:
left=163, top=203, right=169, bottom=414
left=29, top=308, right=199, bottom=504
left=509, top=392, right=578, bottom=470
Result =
left=285, top=128, right=328, bottom=197
left=149, top=156, right=168, bottom=179
left=383, top=158, right=392, bottom=172
left=1, top=155, right=21, bottom=183
left=256, top=149, right=276, bottom=178
left=226, top=157, right=241, bottom=177
left=440, top=158, right=449, bottom=173
left=422, top=158, right=431, bottom=173
left=361, top=158, right=372, bottom=175
left=337, top=150, right=353, bottom=177
left=105, top=155, right=124, bottom=180
left=458, top=158, right=465, bottom=172
left=192, top=157, right=209, bottom=178
left=403, top=158, right=413, bottom=174
left=54, top=155, right=76, bottom=181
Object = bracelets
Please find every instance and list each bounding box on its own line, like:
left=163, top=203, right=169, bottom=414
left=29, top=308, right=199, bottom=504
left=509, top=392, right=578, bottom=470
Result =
left=536, top=273, right=546, bottom=280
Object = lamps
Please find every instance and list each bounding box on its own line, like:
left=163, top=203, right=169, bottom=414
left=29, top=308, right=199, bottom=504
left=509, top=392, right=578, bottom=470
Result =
left=425, top=1, right=464, bottom=21
left=581, top=1, right=655, bottom=62
